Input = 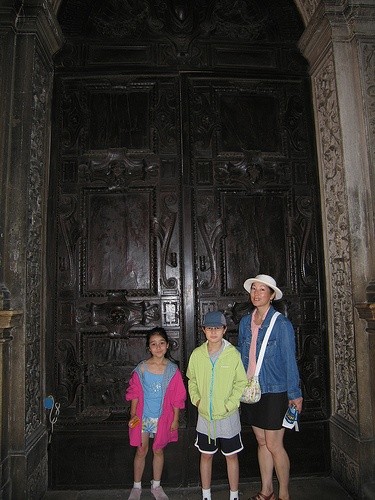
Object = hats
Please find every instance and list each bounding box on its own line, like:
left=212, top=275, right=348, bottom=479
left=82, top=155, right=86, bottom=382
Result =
left=244, top=274, right=282, bottom=300
left=198, top=312, right=226, bottom=328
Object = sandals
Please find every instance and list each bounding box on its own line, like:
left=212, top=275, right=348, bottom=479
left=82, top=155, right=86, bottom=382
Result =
left=246, top=491, right=276, bottom=500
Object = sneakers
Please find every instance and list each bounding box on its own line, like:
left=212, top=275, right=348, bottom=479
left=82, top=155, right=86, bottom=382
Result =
left=128, top=486, right=142, bottom=500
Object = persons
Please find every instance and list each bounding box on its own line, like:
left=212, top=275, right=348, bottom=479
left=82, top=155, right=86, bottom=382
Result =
left=236, top=274, right=303, bottom=500
left=125, top=327, right=185, bottom=500
left=189, top=311, right=247, bottom=500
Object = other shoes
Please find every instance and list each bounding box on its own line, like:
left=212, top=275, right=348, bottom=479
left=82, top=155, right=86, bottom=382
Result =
left=151, top=485, right=168, bottom=500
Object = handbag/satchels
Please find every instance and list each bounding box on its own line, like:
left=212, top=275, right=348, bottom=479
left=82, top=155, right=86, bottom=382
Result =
left=239, top=377, right=262, bottom=404
